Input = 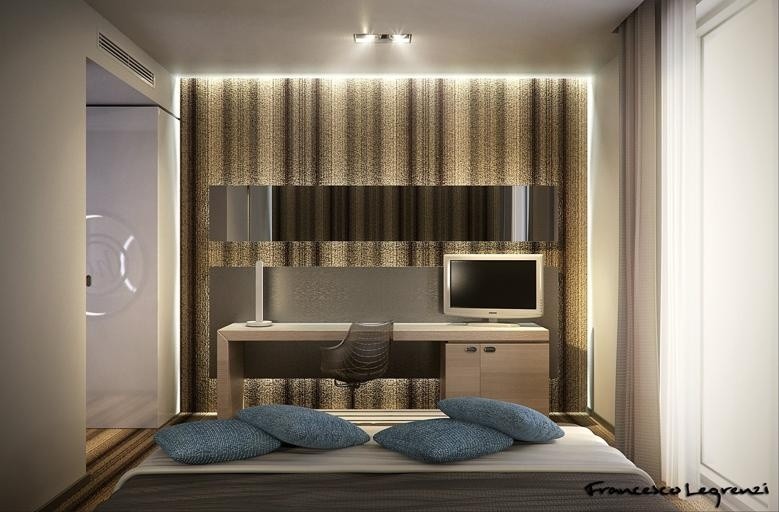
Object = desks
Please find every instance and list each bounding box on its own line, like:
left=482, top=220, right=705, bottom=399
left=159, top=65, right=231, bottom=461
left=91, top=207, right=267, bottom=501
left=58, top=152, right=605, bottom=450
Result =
left=216, top=321, right=549, bottom=422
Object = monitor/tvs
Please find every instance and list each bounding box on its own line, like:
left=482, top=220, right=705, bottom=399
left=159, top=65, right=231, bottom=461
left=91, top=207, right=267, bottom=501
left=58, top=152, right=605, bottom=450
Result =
left=443, top=254, right=544, bottom=326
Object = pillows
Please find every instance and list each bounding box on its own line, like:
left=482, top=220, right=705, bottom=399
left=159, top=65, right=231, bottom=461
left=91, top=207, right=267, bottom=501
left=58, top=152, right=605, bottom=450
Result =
left=154, top=398, right=564, bottom=463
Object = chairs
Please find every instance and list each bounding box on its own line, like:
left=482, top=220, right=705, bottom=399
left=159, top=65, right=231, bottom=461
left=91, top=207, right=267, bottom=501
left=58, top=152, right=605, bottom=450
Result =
left=320, top=319, right=395, bottom=389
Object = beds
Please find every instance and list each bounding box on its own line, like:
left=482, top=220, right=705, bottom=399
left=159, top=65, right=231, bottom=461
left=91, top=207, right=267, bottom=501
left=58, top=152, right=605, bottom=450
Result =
left=89, top=407, right=682, bottom=512
